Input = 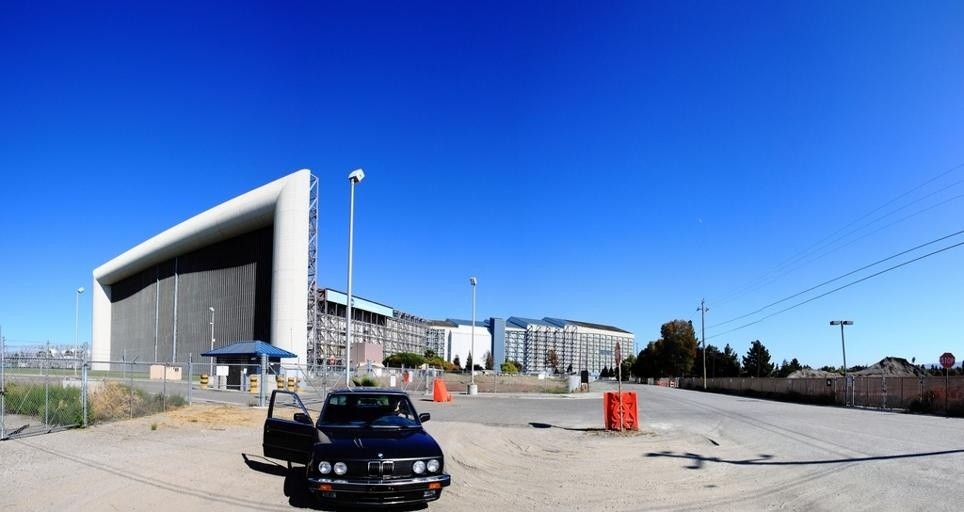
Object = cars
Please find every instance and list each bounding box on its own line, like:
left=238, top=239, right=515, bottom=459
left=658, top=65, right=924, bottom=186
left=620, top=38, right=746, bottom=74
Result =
left=266, top=386, right=452, bottom=506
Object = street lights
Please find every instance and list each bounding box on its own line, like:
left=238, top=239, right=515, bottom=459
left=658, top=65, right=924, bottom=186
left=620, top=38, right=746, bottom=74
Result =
left=697, top=298, right=711, bottom=389
left=468, top=275, right=478, bottom=393
left=208, top=305, right=216, bottom=374
left=74, top=286, right=85, bottom=376
left=347, top=168, right=365, bottom=385
left=830, top=318, right=853, bottom=406
